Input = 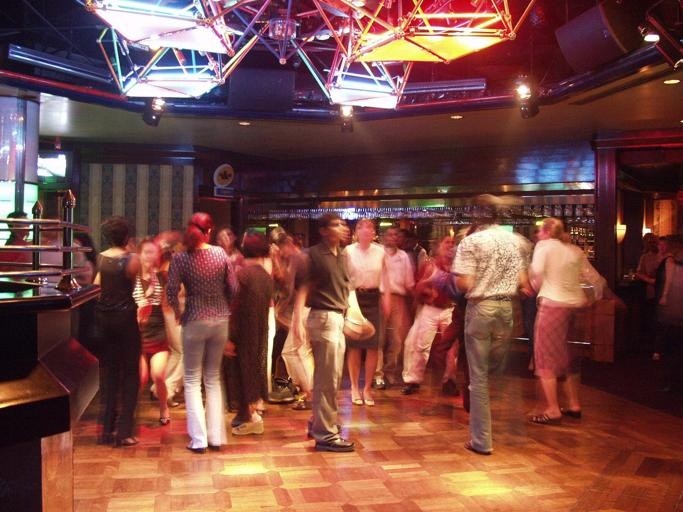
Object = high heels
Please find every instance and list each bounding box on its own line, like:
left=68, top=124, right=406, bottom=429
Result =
left=529, top=411, right=562, bottom=424
left=232, top=419, right=263, bottom=435
left=561, top=407, right=581, bottom=418
left=98, top=431, right=139, bottom=447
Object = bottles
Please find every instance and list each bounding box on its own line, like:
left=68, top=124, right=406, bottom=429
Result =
left=569, top=227, right=593, bottom=259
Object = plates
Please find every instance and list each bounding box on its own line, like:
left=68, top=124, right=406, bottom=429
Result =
left=213, top=164, right=234, bottom=186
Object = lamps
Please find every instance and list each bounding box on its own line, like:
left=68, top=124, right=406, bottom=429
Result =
left=508, top=72, right=539, bottom=122
left=338, top=102, right=357, bottom=136
left=140, top=96, right=174, bottom=127
left=78, top=0, right=538, bottom=116
left=639, top=16, right=662, bottom=48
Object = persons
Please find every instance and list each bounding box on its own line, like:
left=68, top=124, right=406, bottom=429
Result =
left=634, top=230, right=682, bottom=362
left=93, top=210, right=627, bottom=456
left=0, top=211, right=33, bottom=281
left=40, top=215, right=94, bottom=285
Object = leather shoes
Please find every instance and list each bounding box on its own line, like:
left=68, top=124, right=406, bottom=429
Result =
left=443, top=383, right=460, bottom=396
left=351, top=399, right=363, bottom=405
left=315, top=439, right=354, bottom=451
left=365, top=400, right=375, bottom=406
left=401, top=384, right=419, bottom=394
left=308, top=424, right=341, bottom=439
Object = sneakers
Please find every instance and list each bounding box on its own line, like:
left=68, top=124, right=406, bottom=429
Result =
left=373, top=378, right=388, bottom=389
left=160, top=418, right=170, bottom=424
left=464, top=441, right=489, bottom=454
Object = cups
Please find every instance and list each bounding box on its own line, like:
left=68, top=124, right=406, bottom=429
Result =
left=249, top=202, right=593, bottom=224
